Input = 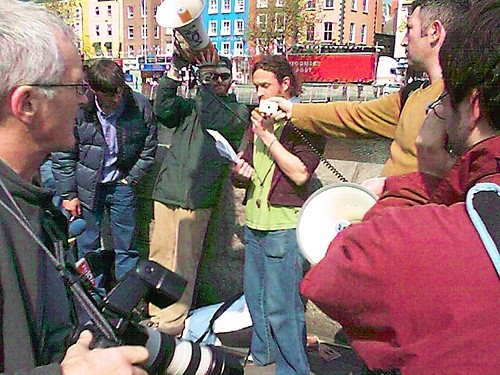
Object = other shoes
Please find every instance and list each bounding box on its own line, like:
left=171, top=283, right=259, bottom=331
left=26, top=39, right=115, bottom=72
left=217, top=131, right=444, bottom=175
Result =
left=239, top=355, right=254, bottom=366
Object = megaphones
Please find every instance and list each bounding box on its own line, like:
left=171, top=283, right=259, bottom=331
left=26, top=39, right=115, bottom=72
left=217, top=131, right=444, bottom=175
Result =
left=156, top=0, right=220, bottom=71
left=296, top=183, right=380, bottom=264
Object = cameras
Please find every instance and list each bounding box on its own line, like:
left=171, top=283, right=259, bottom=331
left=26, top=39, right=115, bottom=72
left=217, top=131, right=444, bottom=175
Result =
left=77, top=258, right=244, bottom=375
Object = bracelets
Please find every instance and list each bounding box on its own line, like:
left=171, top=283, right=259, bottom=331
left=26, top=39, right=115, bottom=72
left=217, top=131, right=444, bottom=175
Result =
left=267, top=139, right=276, bottom=156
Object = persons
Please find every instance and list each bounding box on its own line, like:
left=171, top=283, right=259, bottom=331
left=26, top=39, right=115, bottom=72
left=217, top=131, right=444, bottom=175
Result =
left=299, top=0, right=500, bottom=375
left=149, top=44, right=250, bottom=340
left=51, top=59, right=157, bottom=303
left=229, top=55, right=325, bottom=375
left=0, top=0, right=148, bottom=375
left=255, top=0, right=471, bottom=178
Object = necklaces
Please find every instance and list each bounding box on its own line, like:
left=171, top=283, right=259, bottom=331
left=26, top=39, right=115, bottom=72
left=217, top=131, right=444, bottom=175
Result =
left=254, top=155, right=279, bottom=208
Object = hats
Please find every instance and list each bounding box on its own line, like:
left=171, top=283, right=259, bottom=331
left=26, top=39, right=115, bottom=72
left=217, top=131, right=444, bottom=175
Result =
left=215, top=56, right=232, bottom=69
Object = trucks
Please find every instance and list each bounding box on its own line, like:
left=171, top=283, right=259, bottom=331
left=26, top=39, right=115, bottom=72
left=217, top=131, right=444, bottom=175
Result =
left=288, top=43, right=404, bottom=85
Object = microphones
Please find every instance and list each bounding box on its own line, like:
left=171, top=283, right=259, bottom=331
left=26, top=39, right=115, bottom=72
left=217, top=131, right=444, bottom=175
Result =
left=68, top=219, right=87, bottom=238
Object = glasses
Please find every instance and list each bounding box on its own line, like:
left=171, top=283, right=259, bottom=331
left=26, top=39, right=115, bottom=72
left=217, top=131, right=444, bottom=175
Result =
left=10, top=82, right=90, bottom=96
left=211, top=73, right=231, bottom=80
left=427, top=92, right=448, bottom=120
